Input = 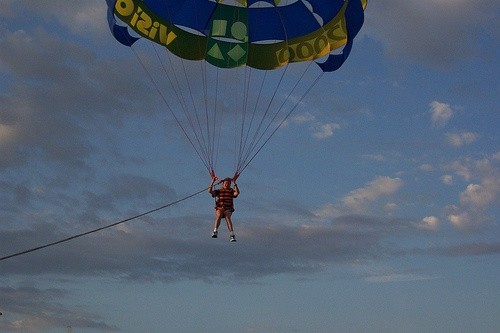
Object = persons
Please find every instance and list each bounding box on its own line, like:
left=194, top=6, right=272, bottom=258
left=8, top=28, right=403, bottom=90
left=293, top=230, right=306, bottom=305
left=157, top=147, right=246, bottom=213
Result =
left=208, top=174, right=240, bottom=242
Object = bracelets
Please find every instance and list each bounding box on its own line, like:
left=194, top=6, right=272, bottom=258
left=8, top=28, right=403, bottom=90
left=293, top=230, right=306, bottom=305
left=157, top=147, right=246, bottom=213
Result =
left=233, top=183, right=237, bottom=185
left=209, top=185, right=212, bottom=188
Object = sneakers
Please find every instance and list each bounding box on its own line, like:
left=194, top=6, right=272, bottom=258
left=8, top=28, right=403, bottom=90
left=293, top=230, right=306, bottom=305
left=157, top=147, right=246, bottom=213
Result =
left=229, top=235, right=236, bottom=242
left=212, top=229, right=218, bottom=238
